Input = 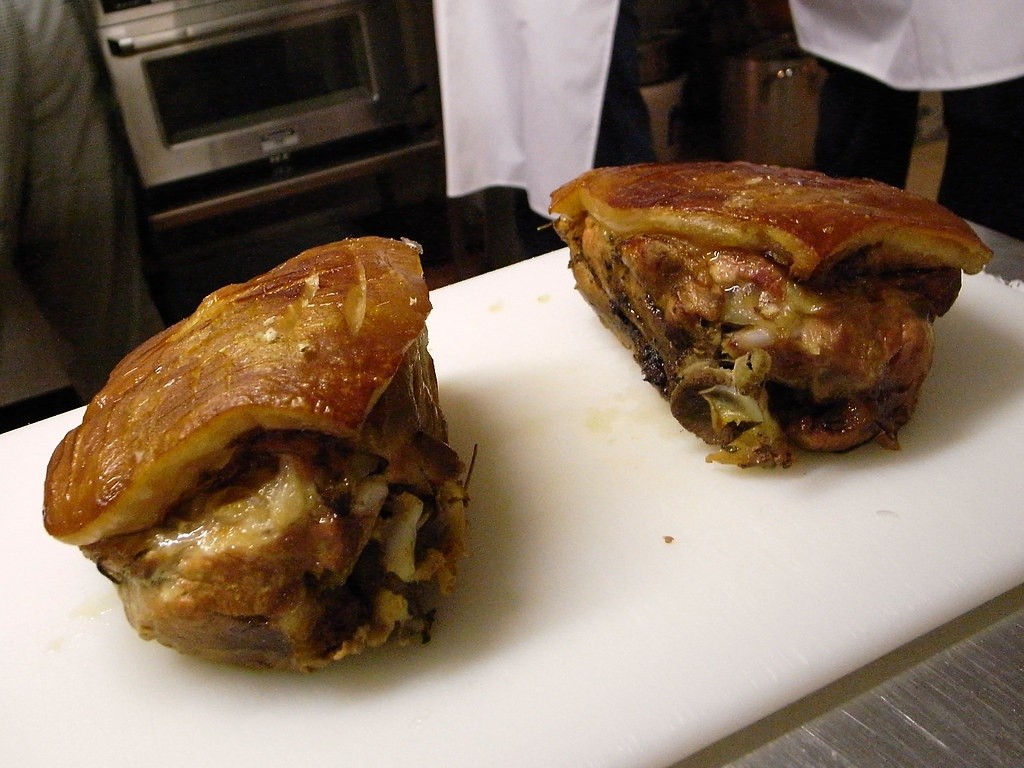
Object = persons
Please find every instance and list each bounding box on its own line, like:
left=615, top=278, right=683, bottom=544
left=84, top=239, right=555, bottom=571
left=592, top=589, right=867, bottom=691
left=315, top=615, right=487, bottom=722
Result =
left=0, top=1, right=162, bottom=435
left=431, top=0, right=1024, bottom=259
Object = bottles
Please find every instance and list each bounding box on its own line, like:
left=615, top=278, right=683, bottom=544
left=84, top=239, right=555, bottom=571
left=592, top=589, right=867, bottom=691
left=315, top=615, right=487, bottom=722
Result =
left=634, top=44, right=685, bottom=163
left=722, top=27, right=817, bottom=169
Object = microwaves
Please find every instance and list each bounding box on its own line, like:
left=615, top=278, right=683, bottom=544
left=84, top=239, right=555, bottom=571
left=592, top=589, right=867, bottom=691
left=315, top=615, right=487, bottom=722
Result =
left=91, top=1, right=441, bottom=189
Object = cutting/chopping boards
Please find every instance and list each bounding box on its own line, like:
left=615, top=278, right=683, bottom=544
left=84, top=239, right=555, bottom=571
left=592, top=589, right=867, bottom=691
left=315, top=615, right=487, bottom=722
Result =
left=0, top=245, right=1024, bottom=766
